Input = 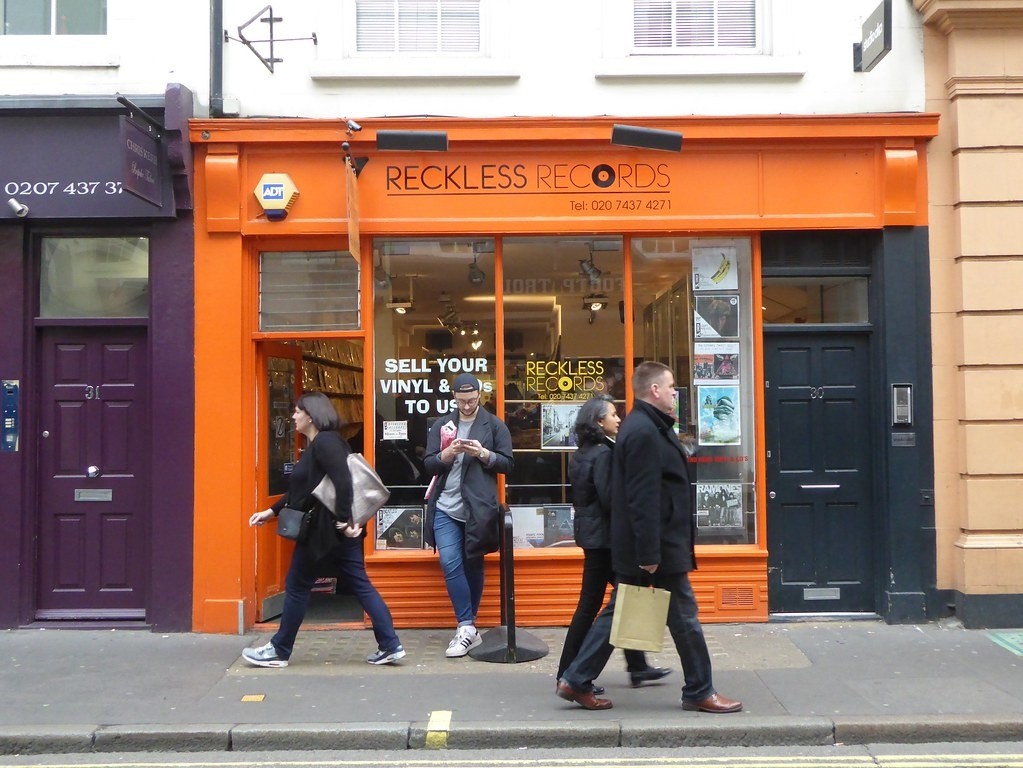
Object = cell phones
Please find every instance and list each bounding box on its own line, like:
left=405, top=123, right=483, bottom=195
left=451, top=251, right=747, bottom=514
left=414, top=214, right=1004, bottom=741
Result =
left=460, top=439, right=471, bottom=446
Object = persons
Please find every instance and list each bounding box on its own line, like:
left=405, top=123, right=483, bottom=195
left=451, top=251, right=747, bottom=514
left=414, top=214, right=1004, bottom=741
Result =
left=704, top=488, right=740, bottom=527
left=586, top=364, right=627, bottom=420
left=707, top=300, right=731, bottom=336
left=556, top=396, right=670, bottom=694
left=483, top=390, right=497, bottom=414
left=241, top=392, right=405, bottom=667
left=387, top=510, right=422, bottom=548
left=556, top=361, right=743, bottom=713
left=424, top=372, right=515, bottom=657
left=518, top=394, right=542, bottom=429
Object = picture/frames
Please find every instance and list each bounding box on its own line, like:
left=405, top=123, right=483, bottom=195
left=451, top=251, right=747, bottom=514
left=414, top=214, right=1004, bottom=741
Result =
left=376, top=504, right=424, bottom=550
left=540, top=403, right=579, bottom=450
left=507, top=504, right=576, bottom=548
left=697, top=386, right=741, bottom=446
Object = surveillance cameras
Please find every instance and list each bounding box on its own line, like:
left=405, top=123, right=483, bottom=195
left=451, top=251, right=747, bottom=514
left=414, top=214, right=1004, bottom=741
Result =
left=348, top=119, right=362, bottom=131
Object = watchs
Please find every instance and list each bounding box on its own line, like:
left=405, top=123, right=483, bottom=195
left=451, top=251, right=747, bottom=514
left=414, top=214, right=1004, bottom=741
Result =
left=479, top=449, right=485, bottom=459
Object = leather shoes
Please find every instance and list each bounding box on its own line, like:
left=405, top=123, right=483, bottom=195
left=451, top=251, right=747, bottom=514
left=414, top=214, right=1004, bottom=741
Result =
left=557, top=683, right=605, bottom=695
left=631, top=665, right=672, bottom=685
left=556, top=680, right=613, bottom=710
left=681, top=691, right=742, bottom=712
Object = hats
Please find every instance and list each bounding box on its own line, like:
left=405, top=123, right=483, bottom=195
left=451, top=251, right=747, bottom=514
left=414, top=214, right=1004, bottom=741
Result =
left=453, top=373, right=480, bottom=393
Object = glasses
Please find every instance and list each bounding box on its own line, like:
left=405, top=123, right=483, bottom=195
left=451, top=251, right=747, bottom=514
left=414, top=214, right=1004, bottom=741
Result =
left=455, top=393, right=480, bottom=407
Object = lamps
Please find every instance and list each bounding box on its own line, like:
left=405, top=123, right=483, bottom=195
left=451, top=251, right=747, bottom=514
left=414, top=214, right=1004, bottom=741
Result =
left=468, top=253, right=484, bottom=285
left=436, top=305, right=460, bottom=335
left=579, top=245, right=600, bottom=281
left=8, top=198, right=28, bottom=217
left=347, top=120, right=360, bottom=136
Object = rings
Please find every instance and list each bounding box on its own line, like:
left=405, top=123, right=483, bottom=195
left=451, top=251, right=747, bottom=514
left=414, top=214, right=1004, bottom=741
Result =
left=475, top=452, right=477, bottom=455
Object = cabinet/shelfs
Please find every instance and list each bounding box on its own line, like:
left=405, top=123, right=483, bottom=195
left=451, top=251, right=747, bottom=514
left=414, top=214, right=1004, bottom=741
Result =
left=300, top=355, right=363, bottom=398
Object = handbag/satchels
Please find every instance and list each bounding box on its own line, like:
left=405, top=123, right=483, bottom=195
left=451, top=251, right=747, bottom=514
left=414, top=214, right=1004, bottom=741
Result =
left=312, top=434, right=391, bottom=528
left=609, top=570, right=672, bottom=653
left=276, top=508, right=312, bottom=540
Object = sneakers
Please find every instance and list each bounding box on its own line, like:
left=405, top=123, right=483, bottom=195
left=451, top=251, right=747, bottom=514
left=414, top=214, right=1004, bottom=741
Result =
left=242, top=641, right=288, bottom=668
left=445, top=624, right=483, bottom=657
left=367, top=644, right=406, bottom=664
